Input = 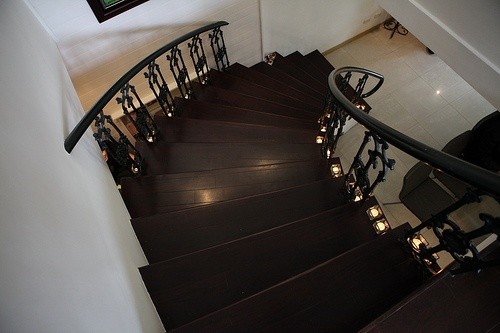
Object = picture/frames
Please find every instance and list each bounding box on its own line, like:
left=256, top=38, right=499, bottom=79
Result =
left=86, top=0, right=152, bottom=24
